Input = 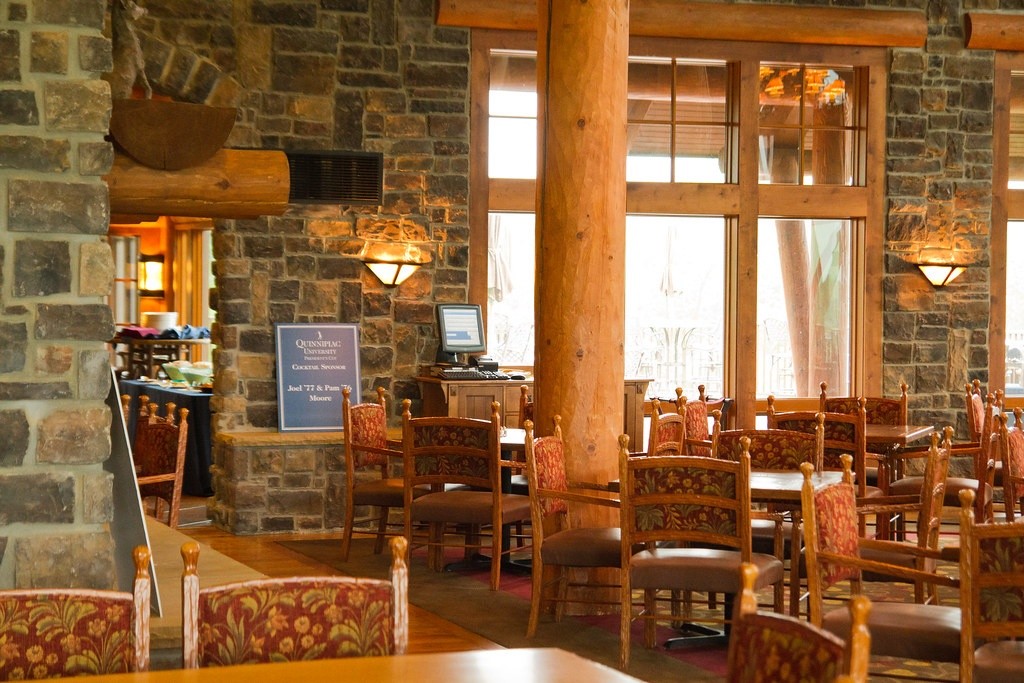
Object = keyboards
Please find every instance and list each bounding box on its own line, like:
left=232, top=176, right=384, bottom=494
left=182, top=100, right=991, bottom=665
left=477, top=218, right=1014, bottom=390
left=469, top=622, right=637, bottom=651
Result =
left=437, top=369, right=511, bottom=380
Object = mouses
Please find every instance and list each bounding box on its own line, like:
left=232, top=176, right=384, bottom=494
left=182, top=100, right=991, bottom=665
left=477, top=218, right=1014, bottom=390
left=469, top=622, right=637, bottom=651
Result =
left=511, top=375, right=526, bottom=380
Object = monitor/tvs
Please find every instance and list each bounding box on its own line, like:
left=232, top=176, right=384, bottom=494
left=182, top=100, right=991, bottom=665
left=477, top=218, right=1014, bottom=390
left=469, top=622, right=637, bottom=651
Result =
left=435, top=302, right=486, bottom=368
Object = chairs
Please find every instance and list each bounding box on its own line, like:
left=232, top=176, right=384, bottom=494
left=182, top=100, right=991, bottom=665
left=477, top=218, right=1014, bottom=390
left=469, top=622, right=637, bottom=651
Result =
left=0, top=372, right=1024, bottom=683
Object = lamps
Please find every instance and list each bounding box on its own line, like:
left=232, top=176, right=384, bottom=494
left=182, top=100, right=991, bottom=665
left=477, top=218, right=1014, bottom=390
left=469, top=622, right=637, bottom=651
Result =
left=914, top=260, right=970, bottom=292
left=359, top=257, right=427, bottom=290
left=136, top=250, right=164, bottom=299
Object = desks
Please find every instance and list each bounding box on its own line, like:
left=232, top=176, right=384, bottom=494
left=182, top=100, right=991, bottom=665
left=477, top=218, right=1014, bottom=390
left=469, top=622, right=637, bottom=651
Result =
left=606, top=468, right=860, bottom=655
left=499, top=427, right=533, bottom=579
left=409, top=374, right=655, bottom=471
left=1, top=646, right=651, bottom=683
left=865, top=421, right=937, bottom=540
left=111, top=338, right=210, bottom=379
left=120, top=378, right=213, bottom=497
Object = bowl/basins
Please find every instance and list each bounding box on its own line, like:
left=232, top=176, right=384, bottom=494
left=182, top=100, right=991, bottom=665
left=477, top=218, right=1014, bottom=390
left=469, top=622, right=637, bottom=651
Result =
left=162, top=363, right=193, bottom=382
left=178, top=368, right=214, bottom=388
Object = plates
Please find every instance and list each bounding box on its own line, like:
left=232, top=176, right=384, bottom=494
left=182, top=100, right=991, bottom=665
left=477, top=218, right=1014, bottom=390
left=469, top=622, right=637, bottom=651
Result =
left=137, top=378, right=187, bottom=388
left=194, top=386, right=212, bottom=393
left=139, top=312, right=178, bottom=335
